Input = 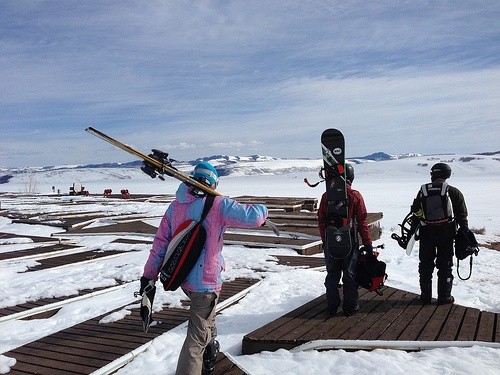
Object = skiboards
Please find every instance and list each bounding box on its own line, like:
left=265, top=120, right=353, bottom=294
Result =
left=84, top=126, right=281, bottom=238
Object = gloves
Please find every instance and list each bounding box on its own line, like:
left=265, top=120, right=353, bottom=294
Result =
left=366, top=245, right=373, bottom=253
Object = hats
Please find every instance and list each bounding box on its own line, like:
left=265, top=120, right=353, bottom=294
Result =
left=192, top=162, right=218, bottom=184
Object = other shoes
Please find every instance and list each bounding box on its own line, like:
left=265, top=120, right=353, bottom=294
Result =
left=328, top=304, right=339, bottom=314
left=437, top=296, right=454, bottom=305
left=421, top=295, right=431, bottom=303
left=344, top=304, right=360, bottom=316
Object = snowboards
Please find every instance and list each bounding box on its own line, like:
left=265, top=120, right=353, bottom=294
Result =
left=320, top=127, right=353, bottom=259
left=405, top=221, right=420, bottom=255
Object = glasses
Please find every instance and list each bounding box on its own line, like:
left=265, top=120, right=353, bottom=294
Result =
left=215, top=178, right=219, bottom=188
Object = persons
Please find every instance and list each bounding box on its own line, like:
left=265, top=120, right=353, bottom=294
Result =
left=407, top=165, right=468, bottom=304
left=139, top=162, right=268, bottom=375
left=317, top=165, right=373, bottom=312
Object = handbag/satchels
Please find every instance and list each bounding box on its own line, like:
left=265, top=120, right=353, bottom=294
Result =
left=358, top=248, right=386, bottom=291
left=159, top=193, right=216, bottom=291
left=456, top=228, right=480, bottom=260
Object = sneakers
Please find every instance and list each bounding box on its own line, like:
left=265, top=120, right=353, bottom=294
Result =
left=204, top=340, right=220, bottom=371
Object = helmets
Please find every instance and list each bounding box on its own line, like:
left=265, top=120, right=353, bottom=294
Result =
left=430, top=163, right=452, bottom=183
left=346, top=164, right=354, bottom=183
